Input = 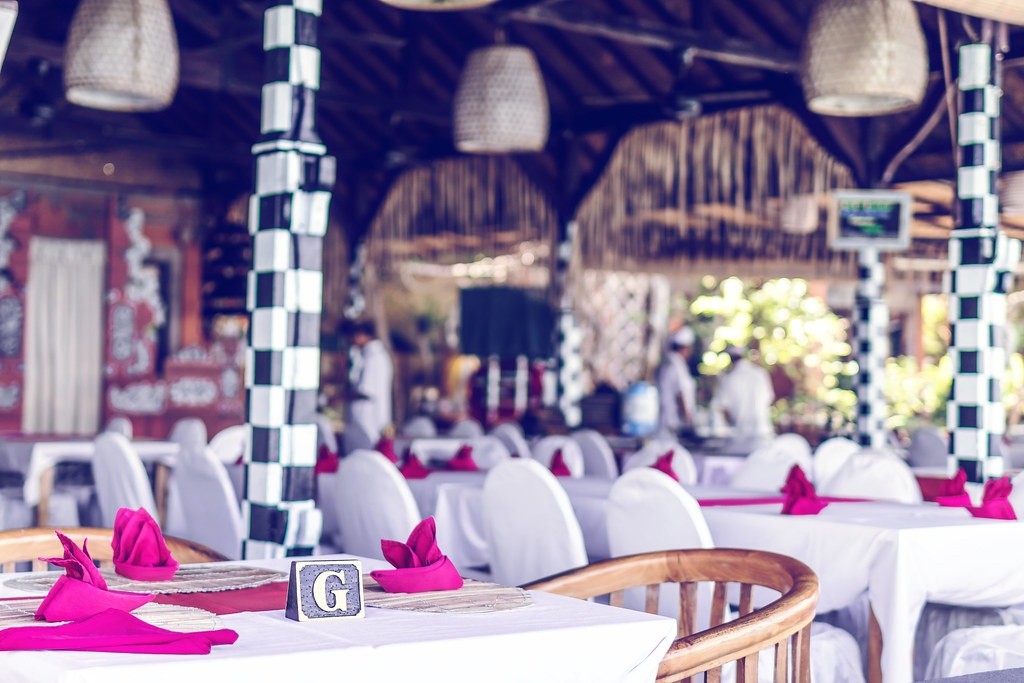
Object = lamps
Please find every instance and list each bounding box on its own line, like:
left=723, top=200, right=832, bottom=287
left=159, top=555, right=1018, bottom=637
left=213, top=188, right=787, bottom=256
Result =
left=803, top=0, right=926, bottom=117
left=455, top=30, right=547, bottom=154
left=63, top=0, right=179, bottom=111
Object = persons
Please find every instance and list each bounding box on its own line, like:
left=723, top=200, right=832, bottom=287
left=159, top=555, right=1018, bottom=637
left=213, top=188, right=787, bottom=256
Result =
left=656, top=325, right=708, bottom=444
left=710, top=344, right=776, bottom=434
left=335, top=318, right=393, bottom=434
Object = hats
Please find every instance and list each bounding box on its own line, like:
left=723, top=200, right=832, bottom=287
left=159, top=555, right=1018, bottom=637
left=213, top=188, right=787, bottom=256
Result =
left=674, top=327, right=695, bottom=347
left=726, top=345, right=744, bottom=356
left=222, top=321, right=240, bottom=337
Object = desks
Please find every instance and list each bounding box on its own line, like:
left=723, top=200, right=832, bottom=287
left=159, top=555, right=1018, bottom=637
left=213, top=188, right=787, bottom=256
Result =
left=5, top=440, right=179, bottom=530
left=0, top=555, right=676, bottom=683
left=676, top=487, right=1024, bottom=683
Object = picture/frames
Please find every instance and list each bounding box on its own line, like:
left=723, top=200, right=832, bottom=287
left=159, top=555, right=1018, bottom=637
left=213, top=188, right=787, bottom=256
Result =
left=825, top=189, right=910, bottom=251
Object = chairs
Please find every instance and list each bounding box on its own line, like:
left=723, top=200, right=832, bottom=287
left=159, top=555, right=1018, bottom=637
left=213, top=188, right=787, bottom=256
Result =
left=0, top=420, right=1024, bottom=683
